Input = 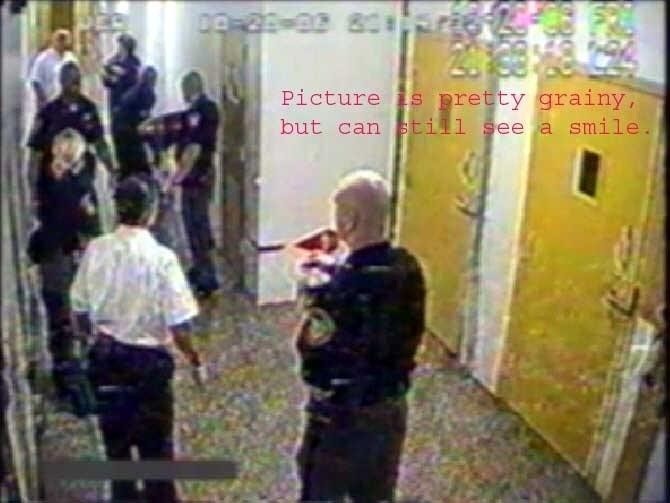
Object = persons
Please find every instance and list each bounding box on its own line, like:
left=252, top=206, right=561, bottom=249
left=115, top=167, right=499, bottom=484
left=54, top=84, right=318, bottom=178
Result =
left=141, top=71, right=221, bottom=292
left=68, top=177, right=206, bottom=502
left=36, top=126, right=99, bottom=405
left=24, top=27, right=156, bottom=184
left=293, top=169, right=426, bottom=502
